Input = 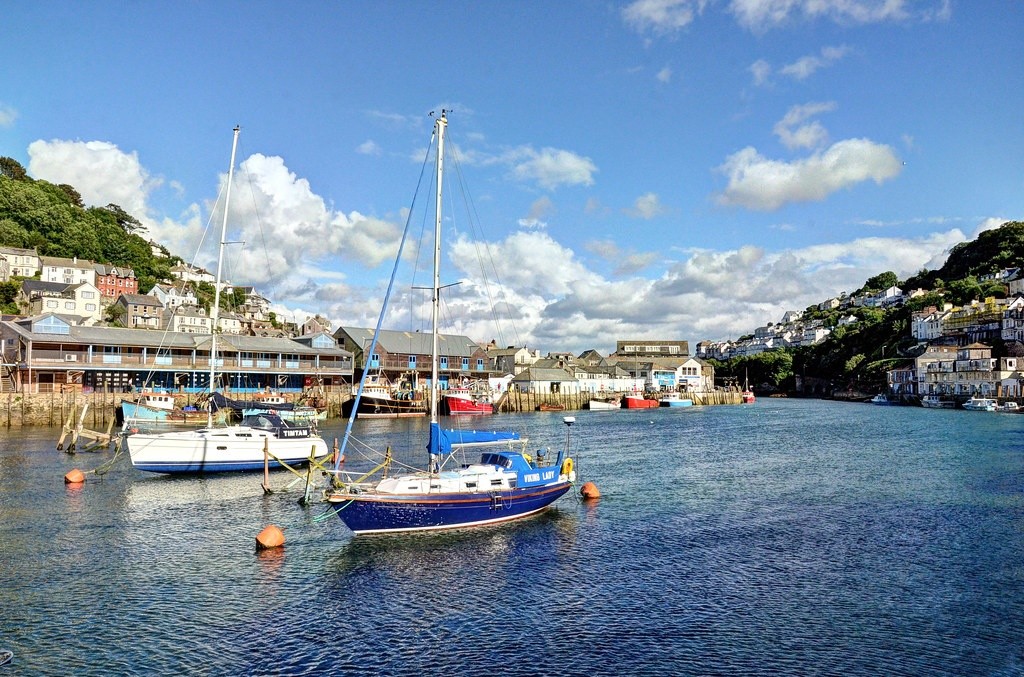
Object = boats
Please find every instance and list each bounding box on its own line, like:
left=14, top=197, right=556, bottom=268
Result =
left=120, top=393, right=234, bottom=430
left=657, top=393, right=693, bottom=408
left=440, top=374, right=494, bottom=415
left=539, top=401, right=564, bottom=411
left=588, top=396, right=622, bottom=411
left=242, top=396, right=328, bottom=424
left=624, top=387, right=660, bottom=408
left=350, top=375, right=427, bottom=418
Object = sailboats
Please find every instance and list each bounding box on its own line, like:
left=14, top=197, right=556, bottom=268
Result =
left=741, top=366, right=756, bottom=404
left=125, top=124, right=329, bottom=476
left=311, top=109, right=581, bottom=538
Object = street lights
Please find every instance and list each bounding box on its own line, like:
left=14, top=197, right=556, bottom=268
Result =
left=634, top=345, right=637, bottom=385
